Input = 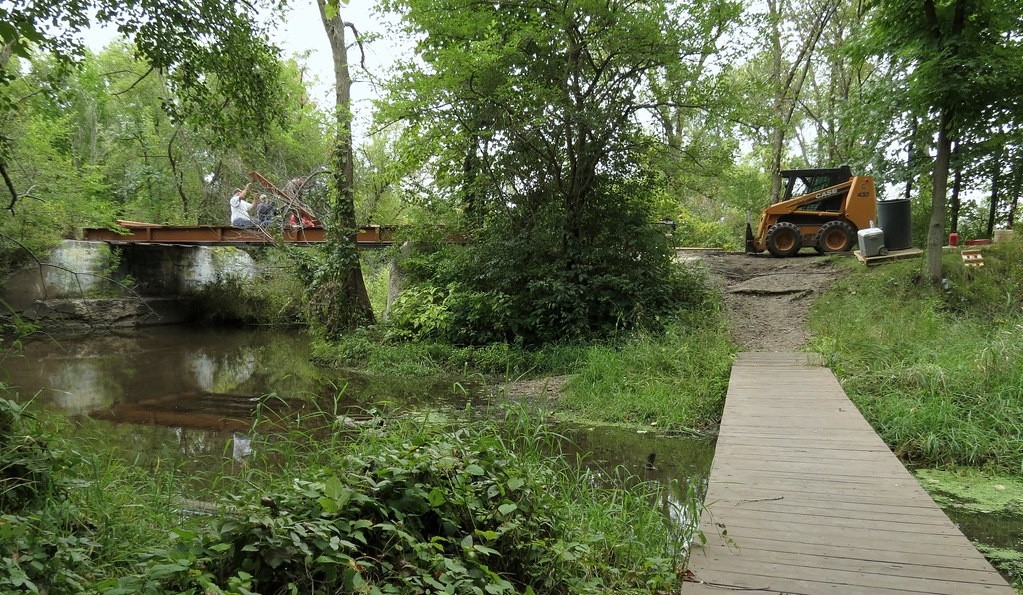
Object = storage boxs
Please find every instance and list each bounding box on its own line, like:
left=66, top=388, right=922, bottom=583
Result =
left=858, top=228, right=888, bottom=257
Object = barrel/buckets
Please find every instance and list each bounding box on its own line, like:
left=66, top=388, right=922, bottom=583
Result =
left=949, top=230, right=957, bottom=246
left=994, top=230, right=1013, bottom=243
left=877, top=198, right=913, bottom=250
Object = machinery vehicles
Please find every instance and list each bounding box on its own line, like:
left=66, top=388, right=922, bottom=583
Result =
left=743, top=167, right=876, bottom=257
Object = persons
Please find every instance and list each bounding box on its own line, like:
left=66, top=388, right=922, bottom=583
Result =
left=280, top=175, right=309, bottom=208
left=229, top=183, right=259, bottom=229
left=253, top=193, right=277, bottom=229
